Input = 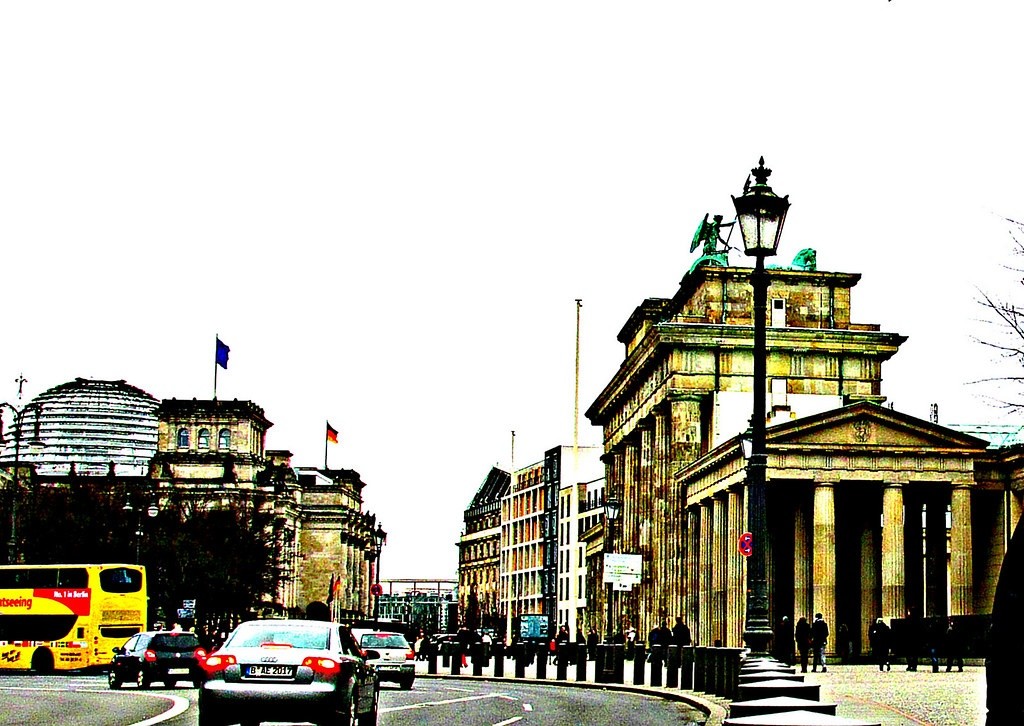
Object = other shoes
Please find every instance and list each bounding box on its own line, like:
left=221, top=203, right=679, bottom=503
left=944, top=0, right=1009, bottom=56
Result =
left=886, top=661, right=890, bottom=671
left=946, top=666, right=951, bottom=672
left=801, top=665, right=827, bottom=673
left=957, top=667, right=963, bottom=672
left=906, top=666, right=911, bottom=671
left=932, top=666, right=939, bottom=673
left=911, top=666, right=917, bottom=671
left=880, top=664, right=884, bottom=672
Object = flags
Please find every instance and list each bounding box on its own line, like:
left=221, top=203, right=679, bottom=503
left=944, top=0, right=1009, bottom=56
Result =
left=216, top=338, right=230, bottom=369
left=327, top=422, right=338, bottom=444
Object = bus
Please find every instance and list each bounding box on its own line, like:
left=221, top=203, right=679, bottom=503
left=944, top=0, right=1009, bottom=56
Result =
left=0, top=563, right=150, bottom=676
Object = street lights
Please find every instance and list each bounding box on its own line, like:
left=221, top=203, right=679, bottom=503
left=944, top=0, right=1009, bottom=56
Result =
left=729, top=157, right=791, bottom=658
left=372, top=522, right=384, bottom=629
left=0, top=401, right=48, bottom=588
left=598, top=490, right=623, bottom=644
left=123, top=491, right=159, bottom=564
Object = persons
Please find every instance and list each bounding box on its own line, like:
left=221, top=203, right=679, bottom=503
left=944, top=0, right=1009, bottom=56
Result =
left=871, top=617, right=890, bottom=671
left=628, top=630, right=636, bottom=642
left=703, top=215, right=736, bottom=253
left=576, top=629, right=588, bottom=661
left=414, top=634, right=438, bottom=661
left=810, top=613, right=829, bottom=672
left=588, top=629, right=598, bottom=661
left=838, top=624, right=849, bottom=665
left=553, top=626, right=568, bottom=665
left=198, top=627, right=224, bottom=651
left=452, top=625, right=492, bottom=667
left=900, top=607, right=921, bottom=671
left=648, top=617, right=690, bottom=667
left=945, top=617, right=963, bottom=672
left=795, top=618, right=811, bottom=672
left=769, top=616, right=795, bottom=668
left=924, top=609, right=943, bottom=673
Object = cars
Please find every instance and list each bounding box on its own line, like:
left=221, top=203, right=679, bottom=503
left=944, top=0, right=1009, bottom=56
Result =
left=198, top=619, right=381, bottom=726
left=108, top=630, right=203, bottom=690
left=349, top=628, right=417, bottom=688
left=431, top=633, right=494, bottom=657
